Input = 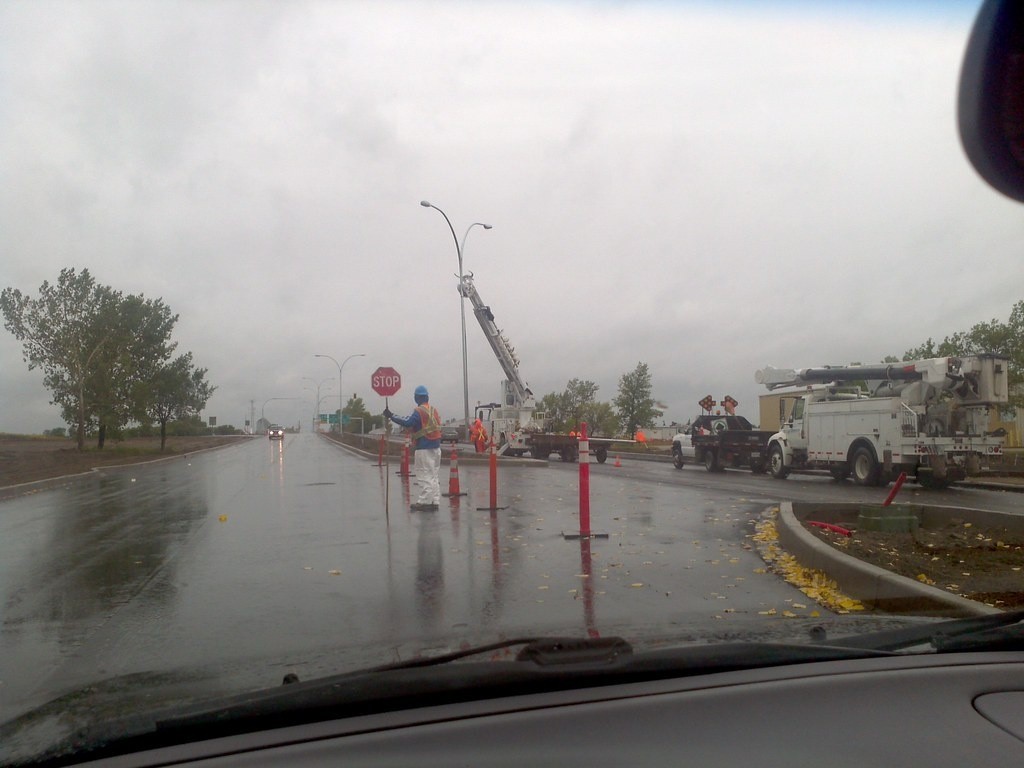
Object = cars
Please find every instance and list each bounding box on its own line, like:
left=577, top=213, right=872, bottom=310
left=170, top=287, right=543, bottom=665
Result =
left=269, top=428, right=284, bottom=438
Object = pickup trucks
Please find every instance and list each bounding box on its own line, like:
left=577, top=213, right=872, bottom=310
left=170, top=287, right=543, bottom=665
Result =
left=672, top=415, right=779, bottom=473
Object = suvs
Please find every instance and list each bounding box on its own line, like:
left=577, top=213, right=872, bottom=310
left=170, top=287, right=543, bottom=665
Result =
left=440, top=426, right=459, bottom=443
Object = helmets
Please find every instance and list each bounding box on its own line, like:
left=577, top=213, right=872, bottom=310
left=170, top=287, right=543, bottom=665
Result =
left=414, top=385, right=428, bottom=395
left=476, top=422, right=481, bottom=427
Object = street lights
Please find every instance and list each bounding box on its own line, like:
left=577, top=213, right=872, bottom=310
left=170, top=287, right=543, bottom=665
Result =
left=421, top=200, right=493, bottom=442
left=303, top=377, right=335, bottom=432
left=315, top=354, right=365, bottom=437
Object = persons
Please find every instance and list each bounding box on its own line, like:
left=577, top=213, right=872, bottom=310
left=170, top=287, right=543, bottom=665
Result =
left=381, top=385, right=442, bottom=512
left=469, top=418, right=488, bottom=455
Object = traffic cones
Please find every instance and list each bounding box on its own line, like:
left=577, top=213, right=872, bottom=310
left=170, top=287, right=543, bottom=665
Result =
left=441, top=449, right=468, bottom=497
left=615, top=455, right=623, bottom=467
left=395, top=444, right=412, bottom=473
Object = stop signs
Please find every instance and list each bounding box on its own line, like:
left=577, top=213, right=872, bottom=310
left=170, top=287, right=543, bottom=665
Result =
left=371, top=367, right=401, bottom=396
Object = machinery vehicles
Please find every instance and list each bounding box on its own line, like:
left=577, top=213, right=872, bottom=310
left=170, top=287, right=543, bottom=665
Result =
left=755, top=354, right=1016, bottom=489
left=453, top=272, right=636, bottom=463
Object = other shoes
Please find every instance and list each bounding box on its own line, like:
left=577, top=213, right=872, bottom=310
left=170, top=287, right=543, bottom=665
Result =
left=410, top=502, right=439, bottom=512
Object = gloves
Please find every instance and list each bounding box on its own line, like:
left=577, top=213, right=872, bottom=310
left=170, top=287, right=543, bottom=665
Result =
left=383, top=409, right=393, bottom=419
